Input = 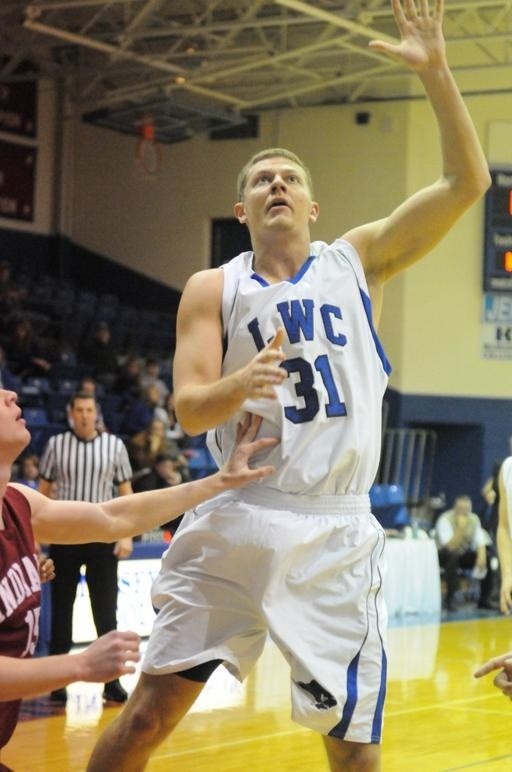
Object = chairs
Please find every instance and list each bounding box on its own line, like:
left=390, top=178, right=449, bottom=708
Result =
left=1, top=365, right=218, bottom=479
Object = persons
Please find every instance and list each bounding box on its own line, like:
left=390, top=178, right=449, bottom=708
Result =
left=84, top=0, right=492, bottom=772
left=0, top=387, right=280, bottom=772
left=472, top=650, right=512, bottom=701
left=394, top=461, right=498, bottom=613
left=36, top=391, right=134, bottom=710
left=495, top=431, right=511, bottom=617
left=0, top=266, right=201, bottom=538
